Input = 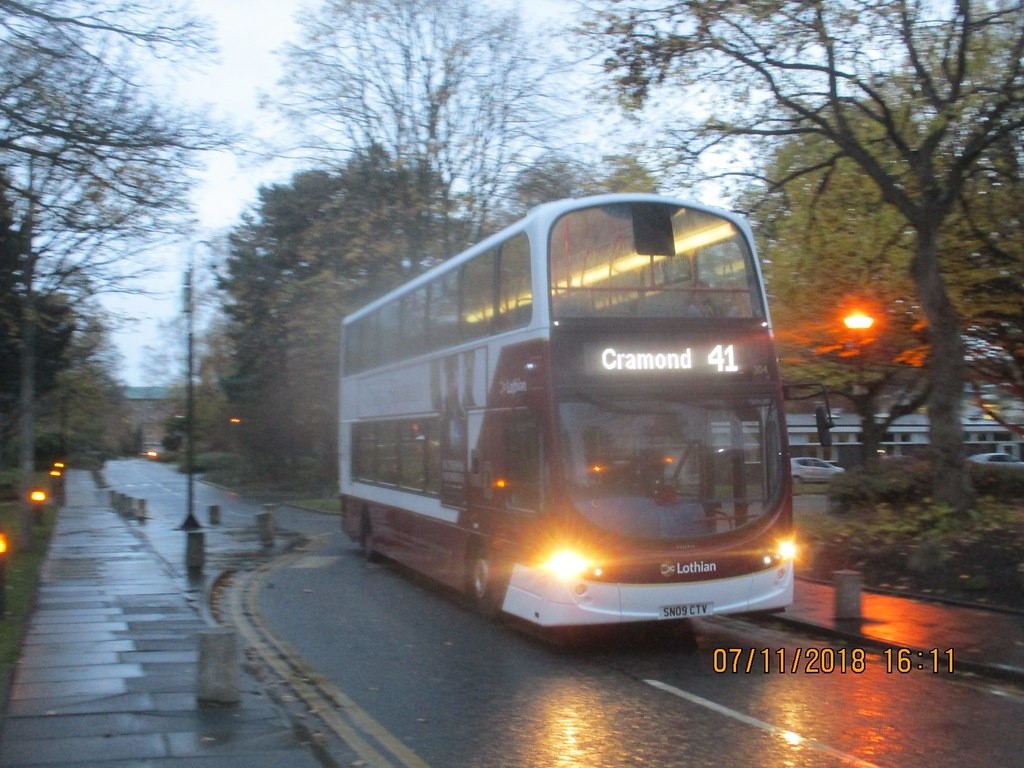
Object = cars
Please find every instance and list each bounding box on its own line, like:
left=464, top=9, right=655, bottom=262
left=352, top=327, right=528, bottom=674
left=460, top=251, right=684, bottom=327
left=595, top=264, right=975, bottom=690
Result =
left=965, top=452, right=1024, bottom=468
left=790, top=457, right=845, bottom=485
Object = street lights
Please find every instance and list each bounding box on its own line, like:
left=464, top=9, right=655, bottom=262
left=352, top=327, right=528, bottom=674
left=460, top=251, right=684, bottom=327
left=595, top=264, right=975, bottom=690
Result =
left=179, top=237, right=218, bottom=532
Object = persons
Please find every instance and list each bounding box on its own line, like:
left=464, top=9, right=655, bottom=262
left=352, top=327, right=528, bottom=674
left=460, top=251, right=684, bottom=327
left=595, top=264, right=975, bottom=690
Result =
left=633, top=275, right=715, bottom=319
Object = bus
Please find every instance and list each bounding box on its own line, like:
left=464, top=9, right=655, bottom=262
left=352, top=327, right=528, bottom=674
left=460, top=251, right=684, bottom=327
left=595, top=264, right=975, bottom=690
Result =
left=338, top=193, right=835, bottom=644
